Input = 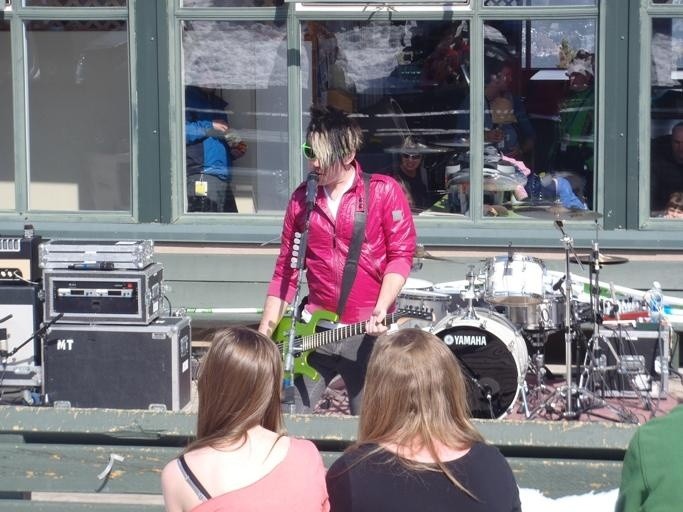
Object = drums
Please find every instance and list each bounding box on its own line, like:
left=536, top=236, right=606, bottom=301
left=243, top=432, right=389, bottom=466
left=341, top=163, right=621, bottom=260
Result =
left=395, top=278, right=495, bottom=331
left=431, top=307, right=530, bottom=419
left=485, top=254, right=544, bottom=308
left=504, top=293, right=578, bottom=333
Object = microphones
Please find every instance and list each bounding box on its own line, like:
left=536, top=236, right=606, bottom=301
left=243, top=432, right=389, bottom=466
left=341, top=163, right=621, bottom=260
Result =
left=305, top=171, right=320, bottom=213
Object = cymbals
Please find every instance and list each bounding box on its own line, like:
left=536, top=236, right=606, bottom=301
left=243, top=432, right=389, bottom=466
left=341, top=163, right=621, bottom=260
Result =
left=413, top=252, right=467, bottom=264
left=569, top=255, right=629, bottom=265
left=513, top=202, right=604, bottom=221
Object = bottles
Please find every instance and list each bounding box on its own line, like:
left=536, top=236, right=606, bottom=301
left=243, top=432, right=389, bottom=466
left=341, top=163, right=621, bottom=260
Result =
left=651, top=280, right=663, bottom=320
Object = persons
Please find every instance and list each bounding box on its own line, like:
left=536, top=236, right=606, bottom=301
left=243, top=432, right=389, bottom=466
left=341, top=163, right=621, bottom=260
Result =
left=159, top=323, right=333, bottom=512
left=381, top=18, right=595, bottom=213
left=180, top=55, right=248, bottom=213
left=256, top=110, right=417, bottom=417
left=649, top=120, right=683, bottom=219
left=614, top=401, right=683, bottom=512
left=324, top=327, right=524, bottom=512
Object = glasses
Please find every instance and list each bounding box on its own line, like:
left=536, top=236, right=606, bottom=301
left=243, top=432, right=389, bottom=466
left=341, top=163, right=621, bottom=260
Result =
left=302, top=142, right=349, bottom=165
left=403, top=152, right=421, bottom=159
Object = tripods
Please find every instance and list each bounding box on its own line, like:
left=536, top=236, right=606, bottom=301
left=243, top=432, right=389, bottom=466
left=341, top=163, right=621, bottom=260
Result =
left=517, top=332, right=560, bottom=417
left=576, top=247, right=651, bottom=417
left=527, top=220, right=639, bottom=425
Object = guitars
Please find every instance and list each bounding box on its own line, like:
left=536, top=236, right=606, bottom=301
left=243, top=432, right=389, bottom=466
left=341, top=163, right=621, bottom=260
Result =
left=269, top=305, right=436, bottom=381
